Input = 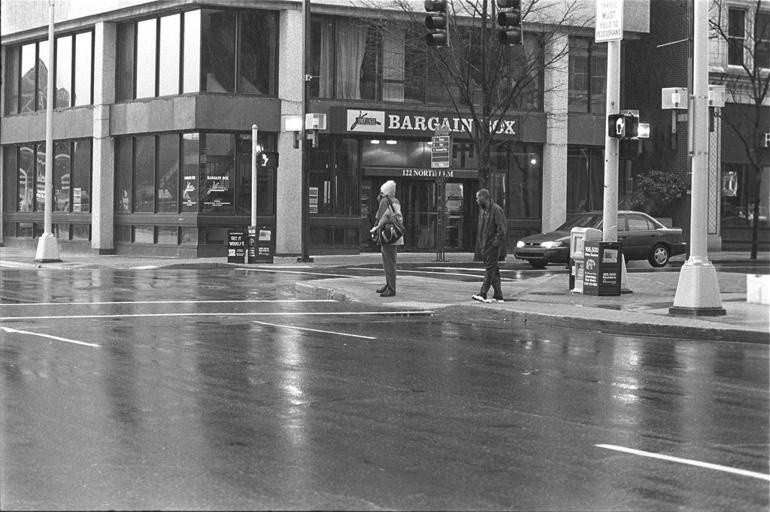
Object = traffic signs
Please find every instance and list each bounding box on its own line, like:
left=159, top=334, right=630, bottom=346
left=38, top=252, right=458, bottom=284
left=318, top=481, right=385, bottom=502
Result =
left=431, top=142, right=449, bottom=146
left=431, top=147, right=448, bottom=152
left=431, top=136, right=449, bottom=141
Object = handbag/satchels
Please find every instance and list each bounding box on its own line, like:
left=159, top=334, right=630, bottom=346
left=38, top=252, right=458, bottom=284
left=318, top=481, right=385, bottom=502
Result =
left=370, top=195, right=406, bottom=246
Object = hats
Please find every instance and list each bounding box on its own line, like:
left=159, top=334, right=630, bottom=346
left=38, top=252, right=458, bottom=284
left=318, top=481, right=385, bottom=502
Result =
left=380, top=180, right=396, bottom=196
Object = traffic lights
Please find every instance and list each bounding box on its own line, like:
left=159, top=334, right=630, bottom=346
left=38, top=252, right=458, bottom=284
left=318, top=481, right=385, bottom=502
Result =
left=257, top=153, right=279, bottom=167
left=496, top=0, right=522, bottom=45
left=608, top=116, right=627, bottom=136
left=425, top=0, right=448, bottom=49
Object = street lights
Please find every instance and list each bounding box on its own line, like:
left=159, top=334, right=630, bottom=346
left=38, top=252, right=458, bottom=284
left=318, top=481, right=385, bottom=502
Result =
left=34, top=0, right=63, bottom=262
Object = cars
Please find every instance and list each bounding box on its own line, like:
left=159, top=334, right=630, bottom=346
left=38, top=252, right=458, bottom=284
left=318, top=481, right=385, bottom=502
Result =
left=513, top=210, right=688, bottom=268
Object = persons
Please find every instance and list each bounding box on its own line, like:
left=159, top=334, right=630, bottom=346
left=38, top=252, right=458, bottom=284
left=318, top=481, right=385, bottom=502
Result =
left=367, top=179, right=404, bottom=298
left=471, top=188, right=508, bottom=302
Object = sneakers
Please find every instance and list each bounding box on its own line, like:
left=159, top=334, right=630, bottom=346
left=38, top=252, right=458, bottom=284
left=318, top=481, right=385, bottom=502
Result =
left=472, top=294, right=486, bottom=302
left=486, top=297, right=504, bottom=303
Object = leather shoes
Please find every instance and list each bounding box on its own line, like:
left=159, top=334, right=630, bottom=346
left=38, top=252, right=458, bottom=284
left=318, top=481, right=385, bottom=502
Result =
left=376, top=285, right=388, bottom=293
left=380, top=289, right=395, bottom=296
